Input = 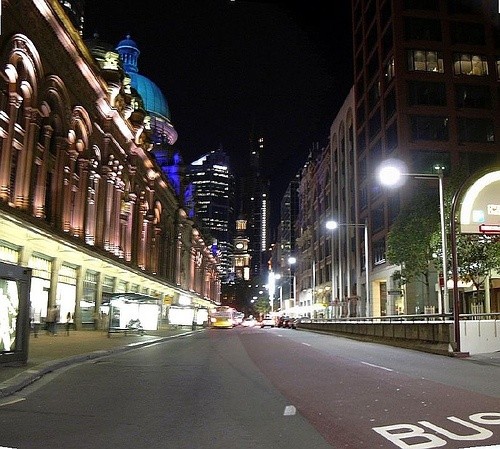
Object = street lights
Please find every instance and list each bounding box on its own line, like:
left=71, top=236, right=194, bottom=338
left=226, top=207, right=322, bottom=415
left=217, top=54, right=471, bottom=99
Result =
left=380, top=166, right=450, bottom=321
left=288, top=258, right=316, bottom=322
left=325, top=221, right=371, bottom=321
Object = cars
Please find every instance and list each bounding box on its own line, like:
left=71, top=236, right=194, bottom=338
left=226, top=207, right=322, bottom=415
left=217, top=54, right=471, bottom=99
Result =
left=260, top=318, right=275, bottom=328
left=277, top=317, right=312, bottom=329
left=242, top=318, right=255, bottom=327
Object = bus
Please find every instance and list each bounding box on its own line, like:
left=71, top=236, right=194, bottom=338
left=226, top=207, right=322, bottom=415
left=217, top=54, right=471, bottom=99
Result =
left=211, top=306, right=243, bottom=329
